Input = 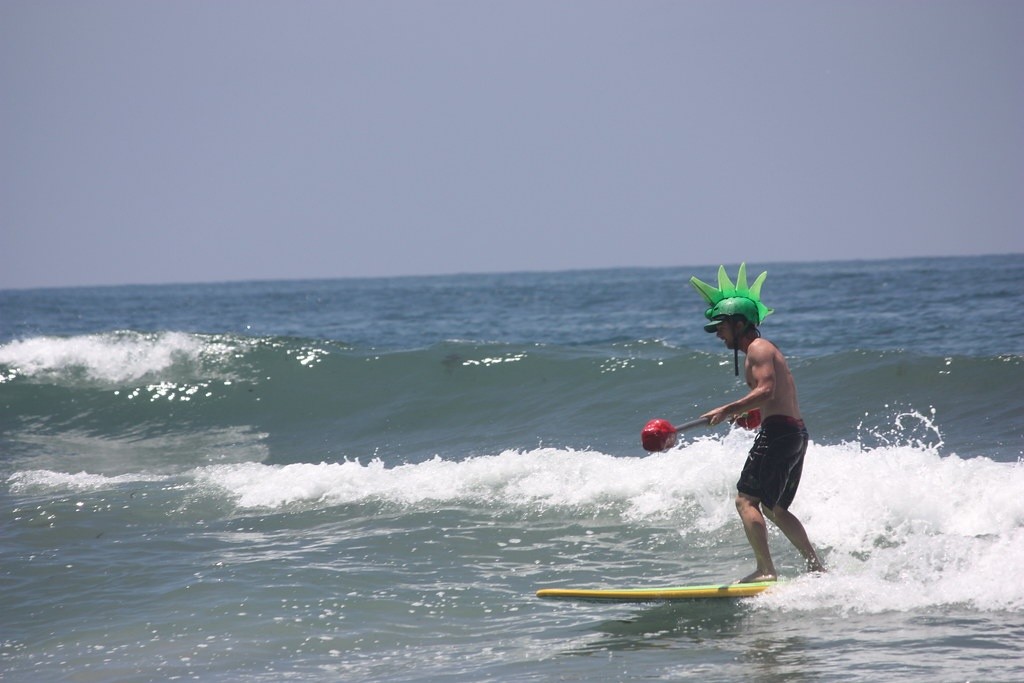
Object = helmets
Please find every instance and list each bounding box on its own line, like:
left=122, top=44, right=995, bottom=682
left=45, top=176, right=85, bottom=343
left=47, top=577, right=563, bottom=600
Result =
left=689, top=263, right=775, bottom=333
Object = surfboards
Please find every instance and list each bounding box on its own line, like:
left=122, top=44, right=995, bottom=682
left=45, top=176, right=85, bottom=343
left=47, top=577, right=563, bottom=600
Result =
left=534, top=578, right=793, bottom=604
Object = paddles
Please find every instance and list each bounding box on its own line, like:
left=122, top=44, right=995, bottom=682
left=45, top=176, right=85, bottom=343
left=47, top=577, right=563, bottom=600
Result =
left=640, top=407, right=762, bottom=452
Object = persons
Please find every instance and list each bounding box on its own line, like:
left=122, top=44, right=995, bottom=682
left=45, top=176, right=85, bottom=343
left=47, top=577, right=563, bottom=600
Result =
left=689, top=263, right=827, bottom=584
